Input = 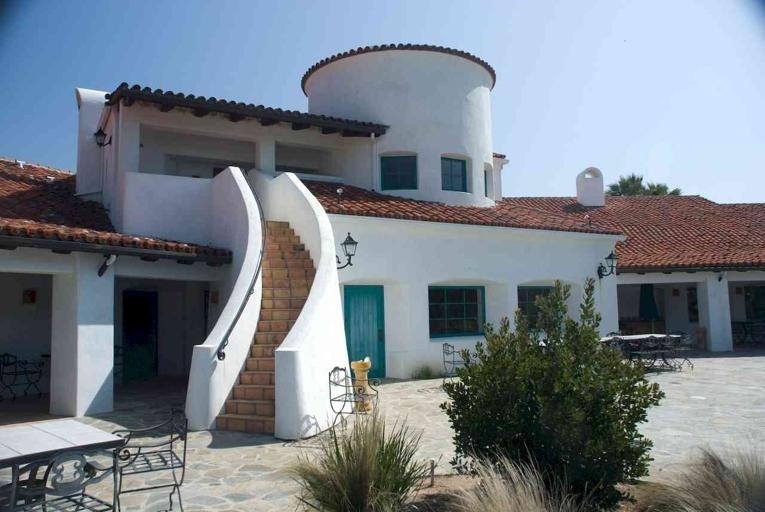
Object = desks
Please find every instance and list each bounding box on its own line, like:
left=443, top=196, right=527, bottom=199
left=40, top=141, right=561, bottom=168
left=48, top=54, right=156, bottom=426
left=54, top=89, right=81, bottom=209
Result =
left=0, top=418, right=126, bottom=512
left=593, top=333, right=682, bottom=368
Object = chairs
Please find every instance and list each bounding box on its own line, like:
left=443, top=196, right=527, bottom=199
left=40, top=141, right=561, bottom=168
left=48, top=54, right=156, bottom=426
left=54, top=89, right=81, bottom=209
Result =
left=732, top=319, right=765, bottom=352
left=0, top=351, right=43, bottom=400
left=442, top=342, right=477, bottom=385
left=329, top=366, right=381, bottom=438
left=109, top=406, right=188, bottom=512
left=605, top=329, right=693, bottom=374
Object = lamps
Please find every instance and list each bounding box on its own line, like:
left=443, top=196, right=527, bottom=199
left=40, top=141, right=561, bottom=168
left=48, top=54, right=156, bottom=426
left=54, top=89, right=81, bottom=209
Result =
left=597, top=250, right=619, bottom=278
left=336, top=232, right=358, bottom=269
left=94, top=126, right=112, bottom=148
